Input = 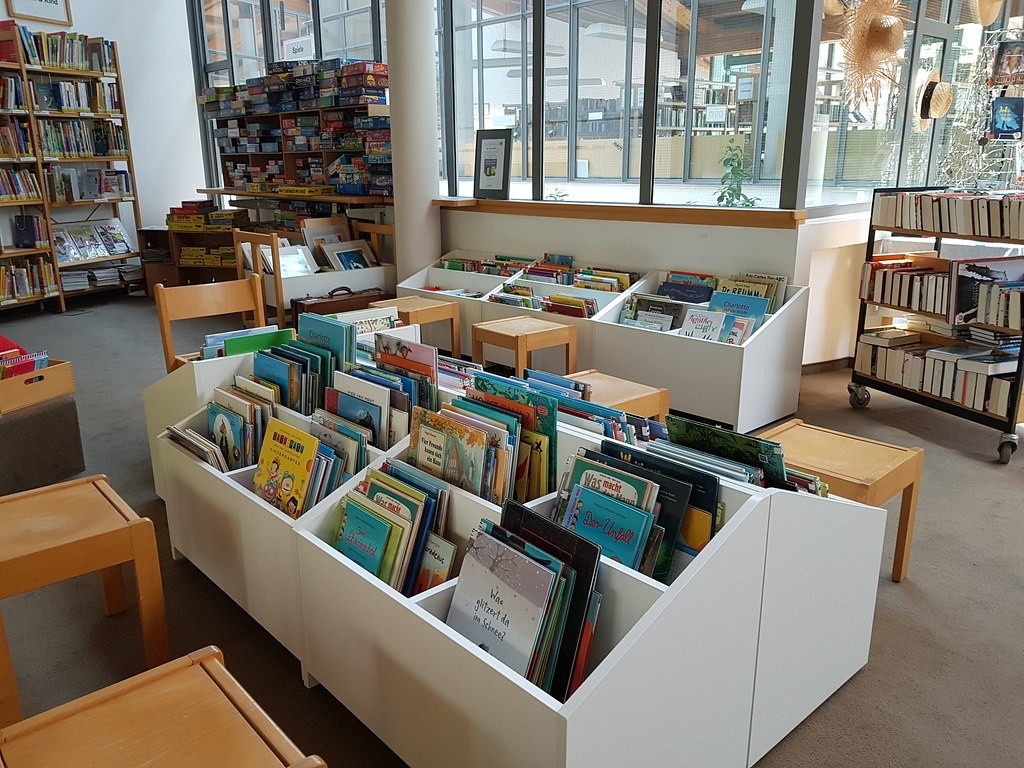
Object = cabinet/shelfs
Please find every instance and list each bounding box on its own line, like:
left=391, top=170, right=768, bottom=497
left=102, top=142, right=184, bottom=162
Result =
left=847, top=185, right=1024, bottom=464
left=504, top=79, right=768, bottom=161
left=0, top=26, right=149, bottom=312
left=137, top=226, right=251, bottom=301
left=209, top=105, right=390, bottom=189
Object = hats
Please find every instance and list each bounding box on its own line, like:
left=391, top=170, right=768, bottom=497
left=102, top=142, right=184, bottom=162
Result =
left=917, top=66, right=953, bottom=132
left=829, top=1, right=916, bottom=108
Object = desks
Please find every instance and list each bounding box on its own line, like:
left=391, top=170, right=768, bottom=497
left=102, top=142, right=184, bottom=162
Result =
left=195, top=188, right=393, bottom=221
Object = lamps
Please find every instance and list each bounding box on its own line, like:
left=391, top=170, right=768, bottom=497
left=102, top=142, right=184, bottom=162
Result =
left=585, top=24, right=664, bottom=43
left=742, top=0, right=825, bottom=20
left=473, top=39, right=606, bottom=87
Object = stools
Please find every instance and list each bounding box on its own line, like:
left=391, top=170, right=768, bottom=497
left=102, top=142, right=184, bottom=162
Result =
left=756, top=418, right=926, bottom=584
left=367, top=294, right=460, bottom=358
left=0, top=474, right=170, bottom=735
left=562, top=369, right=670, bottom=423
left=470, top=314, right=577, bottom=379
left=0, top=645, right=331, bottom=768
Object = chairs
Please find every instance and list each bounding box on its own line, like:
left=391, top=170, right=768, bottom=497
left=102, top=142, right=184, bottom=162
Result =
left=350, top=221, right=395, bottom=264
left=154, top=272, right=266, bottom=375
left=232, top=228, right=287, bottom=328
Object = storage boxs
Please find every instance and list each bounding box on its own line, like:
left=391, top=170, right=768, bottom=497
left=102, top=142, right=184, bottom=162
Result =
left=256, top=198, right=347, bottom=231
left=198, top=58, right=394, bottom=195
left=166, top=200, right=249, bottom=267
left=0, top=360, right=75, bottom=415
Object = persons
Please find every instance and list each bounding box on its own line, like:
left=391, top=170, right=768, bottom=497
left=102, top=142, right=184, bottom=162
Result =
left=999, top=47, right=1024, bottom=75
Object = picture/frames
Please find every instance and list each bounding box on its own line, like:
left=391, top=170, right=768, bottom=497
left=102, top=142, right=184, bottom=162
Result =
left=5, top=0, right=73, bottom=26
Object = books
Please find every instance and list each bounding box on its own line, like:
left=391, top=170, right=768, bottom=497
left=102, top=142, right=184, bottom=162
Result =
left=872, top=192, right=1024, bottom=240
left=165, top=311, right=832, bottom=703
left=990, top=97, right=1024, bottom=140
left=419, top=253, right=788, bottom=345
left=989, top=41, right=1024, bottom=85
left=0, top=23, right=143, bottom=307
left=0, top=348, right=50, bottom=380
left=854, top=251, right=1024, bottom=417
